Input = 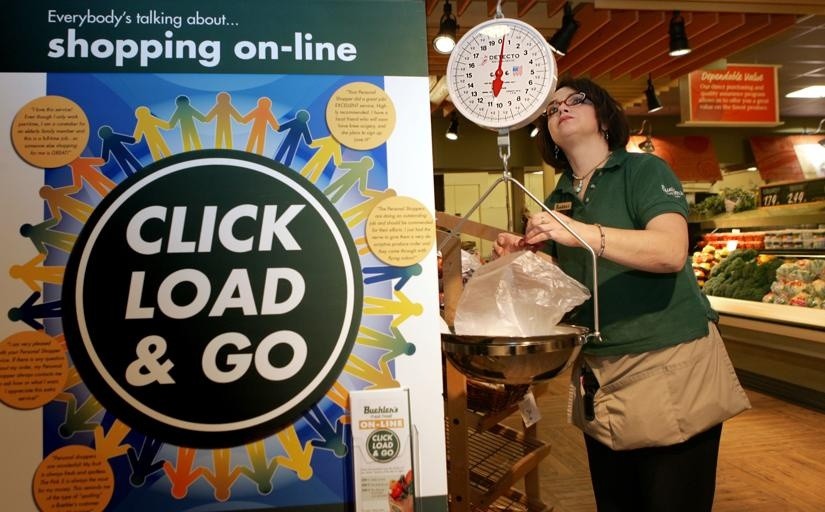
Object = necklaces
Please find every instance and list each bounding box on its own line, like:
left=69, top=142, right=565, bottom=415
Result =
left=571, top=151, right=615, bottom=195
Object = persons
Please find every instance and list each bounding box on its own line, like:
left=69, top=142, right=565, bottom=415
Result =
left=488, top=76, right=726, bottom=511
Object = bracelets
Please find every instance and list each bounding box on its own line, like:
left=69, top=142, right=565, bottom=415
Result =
left=593, top=221, right=605, bottom=261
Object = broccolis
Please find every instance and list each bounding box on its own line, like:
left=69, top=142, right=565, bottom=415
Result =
left=699, top=248, right=783, bottom=302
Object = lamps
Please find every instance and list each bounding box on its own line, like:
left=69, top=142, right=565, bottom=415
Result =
left=431, top=2, right=692, bottom=141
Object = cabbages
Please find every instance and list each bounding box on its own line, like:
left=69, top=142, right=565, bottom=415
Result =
left=763, top=258, right=825, bottom=308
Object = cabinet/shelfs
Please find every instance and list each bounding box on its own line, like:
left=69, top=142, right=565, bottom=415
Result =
left=434, top=212, right=552, bottom=511
left=699, top=200, right=825, bottom=393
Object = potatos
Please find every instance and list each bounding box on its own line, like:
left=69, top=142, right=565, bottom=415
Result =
left=689, top=245, right=729, bottom=288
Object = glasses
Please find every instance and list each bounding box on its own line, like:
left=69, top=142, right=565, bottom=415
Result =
left=540, top=89, right=599, bottom=121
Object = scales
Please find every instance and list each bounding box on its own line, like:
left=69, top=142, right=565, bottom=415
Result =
left=439, top=17, right=599, bottom=386
